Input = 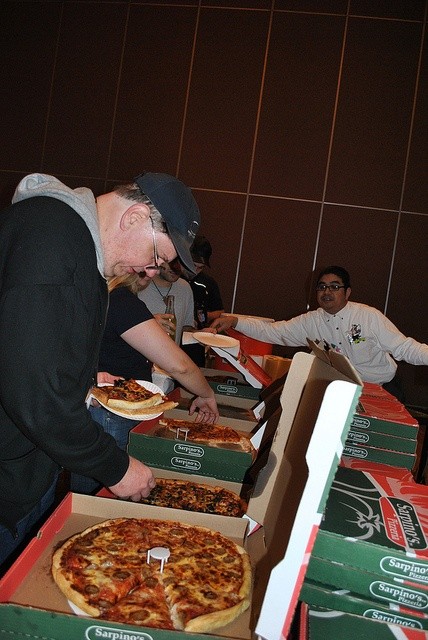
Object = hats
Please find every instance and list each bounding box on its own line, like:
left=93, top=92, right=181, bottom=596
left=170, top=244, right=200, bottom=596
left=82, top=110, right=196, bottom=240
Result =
left=134, top=172, right=200, bottom=275
left=189, top=234, right=211, bottom=269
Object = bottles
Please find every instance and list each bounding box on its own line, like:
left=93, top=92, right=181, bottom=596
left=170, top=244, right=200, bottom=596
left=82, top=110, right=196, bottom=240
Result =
left=163, top=293, right=178, bottom=340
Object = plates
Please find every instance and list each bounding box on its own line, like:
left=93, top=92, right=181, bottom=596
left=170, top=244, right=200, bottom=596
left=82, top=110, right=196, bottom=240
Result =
left=82, top=368, right=172, bottom=419
left=191, top=331, right=240, bottom=348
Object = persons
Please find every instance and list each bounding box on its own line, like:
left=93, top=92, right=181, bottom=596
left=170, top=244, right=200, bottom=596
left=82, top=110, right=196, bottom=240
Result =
left=181, top=234, right=224, bottom=367
left=98, top=269, right=220, bottom=427
left=210, top=266, right=428, bottom=407
left=0, top=172, right=200, bottom=573
left=136, top=256, right=219, bottom=396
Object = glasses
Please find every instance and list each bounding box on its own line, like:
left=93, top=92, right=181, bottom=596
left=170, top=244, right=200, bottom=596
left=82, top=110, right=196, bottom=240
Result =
left=318, top=284, right=344, bottom=291
left=145, top=216, right=166, bottom=271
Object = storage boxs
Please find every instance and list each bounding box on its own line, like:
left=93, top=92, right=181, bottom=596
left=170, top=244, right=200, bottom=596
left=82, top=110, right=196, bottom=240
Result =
left=305, top=555, right=428, bottom=614
left=351, top=382, right=419, bottom=440
left=311, top=466, right=428, bottom=585
left=95, top=445, right=271, bottom=497
left=343, top=443, right=416, bottom=470
left=340, top=455, right=414, bottom=483
left=0, top=337, right=364, bottom=640
left=199, top=346, right=273, bottom=401
left=126, top=405, right=283, bottom=486
left=346, top=428, right=417, bottom=455
left=298, top=580, right=427, bottom=630
left=308, top=606, right=428, bottom=640
left=167, top=385, right=284, bottom=424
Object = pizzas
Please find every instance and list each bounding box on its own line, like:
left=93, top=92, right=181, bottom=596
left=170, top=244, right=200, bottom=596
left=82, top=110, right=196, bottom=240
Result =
left=107, top=377, right=164, bottom=410
left=147, top=476, right=247, bottom=517
left=88, top=387, right=179, bottom=417
left=159, top=417, right=254, bottom=456
left=50, top=515, right=252, bottom=633
